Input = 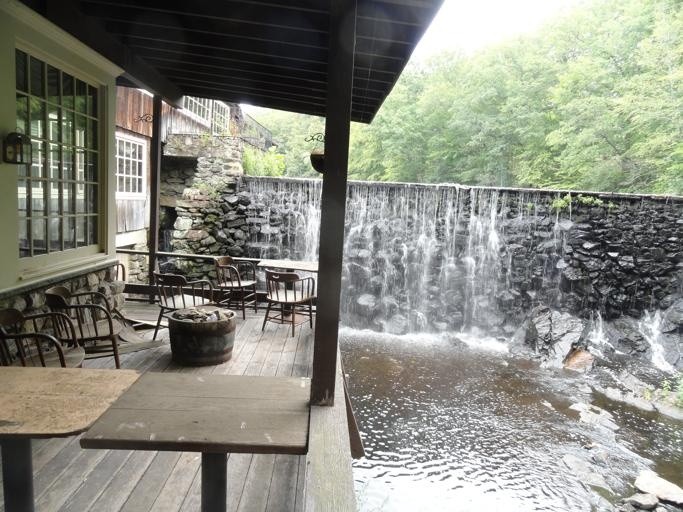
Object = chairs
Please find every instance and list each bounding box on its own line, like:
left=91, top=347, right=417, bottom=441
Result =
left=215, top=254, right=258, bottom=321
left=0, top=306, right=86, bottom=372
left=150, top=269, right=219, bottom=341
left=260, top=267, right=317, bottom=339
left=44, top=283, right=124, bottom=370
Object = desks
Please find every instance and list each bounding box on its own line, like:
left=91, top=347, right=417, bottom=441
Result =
left=0, top=363, right=142, bottom=512
left=78, top=366, right=317, bottom=512
